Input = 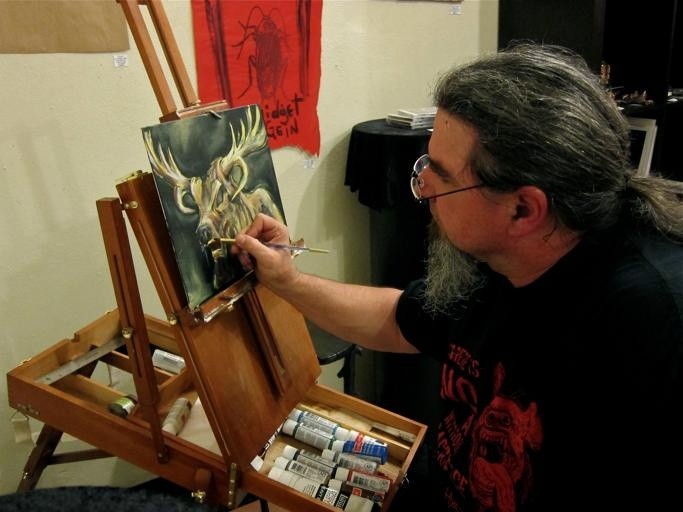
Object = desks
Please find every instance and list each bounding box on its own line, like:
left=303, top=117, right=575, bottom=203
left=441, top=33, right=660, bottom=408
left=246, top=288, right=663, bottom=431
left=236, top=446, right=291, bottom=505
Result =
left=346, top=117, right=441, bottom=493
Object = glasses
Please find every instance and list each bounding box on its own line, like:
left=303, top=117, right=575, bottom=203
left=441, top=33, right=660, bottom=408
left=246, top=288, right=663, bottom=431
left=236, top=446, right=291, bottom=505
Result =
left=411, top=155, right=487, bottom=203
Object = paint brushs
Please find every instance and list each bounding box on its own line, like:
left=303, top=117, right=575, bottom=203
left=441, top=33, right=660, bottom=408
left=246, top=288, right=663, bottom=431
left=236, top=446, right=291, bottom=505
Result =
left=213, top=238, right=329, bottom=254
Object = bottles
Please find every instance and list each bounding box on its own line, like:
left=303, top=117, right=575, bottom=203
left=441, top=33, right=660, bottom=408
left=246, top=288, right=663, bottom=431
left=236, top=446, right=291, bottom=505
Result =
left=276, top=408, right=390, bottom=502
left=109, top=394, right=137, bottom=418
left=161, top=397, right=193, bottom=436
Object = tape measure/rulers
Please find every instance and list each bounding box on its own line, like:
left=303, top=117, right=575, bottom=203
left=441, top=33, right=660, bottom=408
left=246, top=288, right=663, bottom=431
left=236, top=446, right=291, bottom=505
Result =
left=34, top=335, right=124, bottom=385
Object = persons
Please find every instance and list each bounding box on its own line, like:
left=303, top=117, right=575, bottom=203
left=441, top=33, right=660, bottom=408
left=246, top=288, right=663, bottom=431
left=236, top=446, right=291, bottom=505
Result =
left=228, top=40, right=682, bottom=512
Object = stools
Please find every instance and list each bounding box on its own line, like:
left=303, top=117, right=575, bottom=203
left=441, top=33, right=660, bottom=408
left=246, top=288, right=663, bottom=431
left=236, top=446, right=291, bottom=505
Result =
left=303, top=319, right=359, bottom=396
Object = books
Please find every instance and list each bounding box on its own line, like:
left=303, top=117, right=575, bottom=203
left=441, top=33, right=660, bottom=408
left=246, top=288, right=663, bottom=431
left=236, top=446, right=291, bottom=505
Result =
left=385, top=105, right=439, bottom=130
